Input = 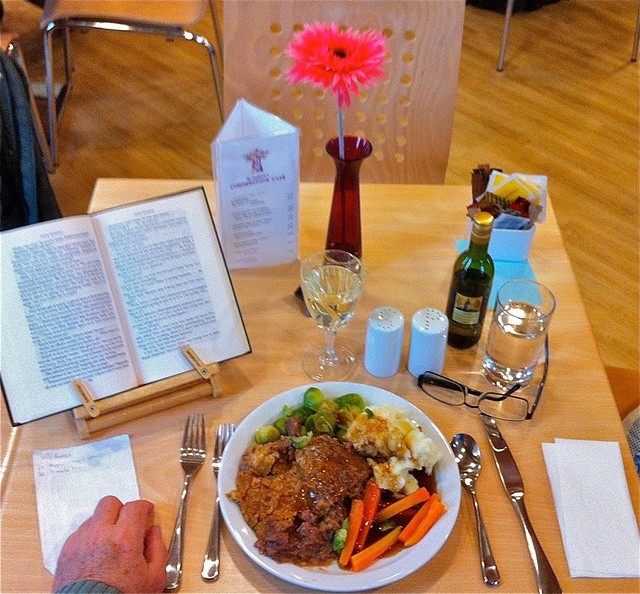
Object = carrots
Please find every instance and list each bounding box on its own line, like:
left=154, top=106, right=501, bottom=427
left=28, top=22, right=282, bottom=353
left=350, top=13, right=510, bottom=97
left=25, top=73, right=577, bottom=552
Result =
left=339, top=479, right=446, bottom=571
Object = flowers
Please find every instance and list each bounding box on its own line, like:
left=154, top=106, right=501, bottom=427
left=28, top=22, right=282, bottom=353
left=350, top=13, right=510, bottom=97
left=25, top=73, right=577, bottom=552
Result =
left=285, top=20, right=393, bottom=258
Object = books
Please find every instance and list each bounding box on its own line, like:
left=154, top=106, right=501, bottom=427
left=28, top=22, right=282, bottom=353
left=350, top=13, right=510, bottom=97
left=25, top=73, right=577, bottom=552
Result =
left=0, top=184, right=253, bottom=427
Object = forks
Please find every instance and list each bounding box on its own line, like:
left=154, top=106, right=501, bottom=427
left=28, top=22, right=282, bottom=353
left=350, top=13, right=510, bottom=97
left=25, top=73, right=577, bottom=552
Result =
left=201, top=422, right=237, bottom=581
left=163, top=412, right=207, bottom=590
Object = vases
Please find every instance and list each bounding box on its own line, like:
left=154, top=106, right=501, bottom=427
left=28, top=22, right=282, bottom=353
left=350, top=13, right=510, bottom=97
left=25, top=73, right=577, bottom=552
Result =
left=322, top=134, right=374, bottom=271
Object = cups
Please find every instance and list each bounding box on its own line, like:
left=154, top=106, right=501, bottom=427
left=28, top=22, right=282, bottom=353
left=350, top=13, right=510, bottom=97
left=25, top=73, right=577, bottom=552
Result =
left=482, top=278, right=557, bottom=388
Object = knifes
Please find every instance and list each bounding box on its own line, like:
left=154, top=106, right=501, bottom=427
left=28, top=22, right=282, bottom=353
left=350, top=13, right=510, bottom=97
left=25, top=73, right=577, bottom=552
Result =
left=480, top=413, right=561, bottom=593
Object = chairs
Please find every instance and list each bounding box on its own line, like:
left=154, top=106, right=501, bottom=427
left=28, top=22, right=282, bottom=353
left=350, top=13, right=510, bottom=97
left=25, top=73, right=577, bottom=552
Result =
left=38, top=0, right=226, bottom=166
left=1, top=0, right=55, bottom=173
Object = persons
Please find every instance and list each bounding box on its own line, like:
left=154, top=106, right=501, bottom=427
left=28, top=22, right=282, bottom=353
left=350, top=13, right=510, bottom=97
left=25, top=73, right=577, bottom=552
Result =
left=51, top=494, right=168, bottom=594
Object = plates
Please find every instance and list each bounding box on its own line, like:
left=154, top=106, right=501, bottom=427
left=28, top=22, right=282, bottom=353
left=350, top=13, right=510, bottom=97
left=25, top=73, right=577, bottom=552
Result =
left=217, top=381, right=462, bottom=593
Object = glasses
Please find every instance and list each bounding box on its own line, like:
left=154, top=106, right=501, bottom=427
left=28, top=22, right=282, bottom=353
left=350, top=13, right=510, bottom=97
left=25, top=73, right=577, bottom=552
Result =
left=417, top=333, right=549, bottom=422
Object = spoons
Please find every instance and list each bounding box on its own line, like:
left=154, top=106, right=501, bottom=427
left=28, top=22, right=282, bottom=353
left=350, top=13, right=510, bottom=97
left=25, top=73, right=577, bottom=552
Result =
left=449, top=433, right=500, bottom=587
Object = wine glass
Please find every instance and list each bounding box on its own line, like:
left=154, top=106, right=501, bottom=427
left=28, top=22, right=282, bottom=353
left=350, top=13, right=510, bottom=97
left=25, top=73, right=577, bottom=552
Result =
left=299, top=250, right=367, bottom=381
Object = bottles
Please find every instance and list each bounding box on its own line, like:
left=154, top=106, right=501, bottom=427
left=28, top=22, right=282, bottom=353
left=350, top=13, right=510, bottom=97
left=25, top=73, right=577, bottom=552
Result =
left=364, top=306, right=405, bottom=378
left=407, top=308, right=450, bottom=380
left=446, top=211, right=495, bottom=350
